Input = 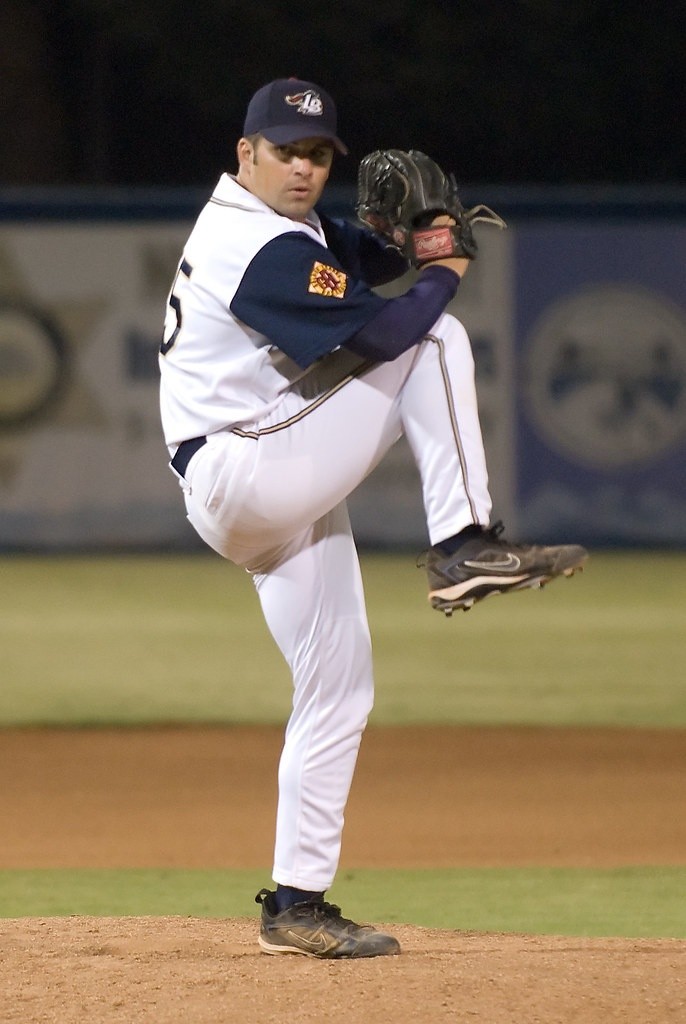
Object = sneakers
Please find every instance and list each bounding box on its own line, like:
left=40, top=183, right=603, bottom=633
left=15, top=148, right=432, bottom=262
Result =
left=424, top=520, right=589, bottom=616
left=254, top=889, right=402, bottom=958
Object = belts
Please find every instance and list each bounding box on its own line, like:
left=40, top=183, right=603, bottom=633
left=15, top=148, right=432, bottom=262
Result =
left=171, top=435, right=206, bottom=478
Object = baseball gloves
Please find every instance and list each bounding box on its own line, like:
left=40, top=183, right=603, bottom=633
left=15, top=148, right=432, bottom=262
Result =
left=356, top=145, right=478, bottom=267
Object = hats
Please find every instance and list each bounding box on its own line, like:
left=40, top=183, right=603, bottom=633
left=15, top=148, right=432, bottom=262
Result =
left=243, top=77, right=349, bottom=156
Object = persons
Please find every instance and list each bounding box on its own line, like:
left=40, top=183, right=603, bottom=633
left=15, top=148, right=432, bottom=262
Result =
left=155, top=77, right=588, bottom=962
